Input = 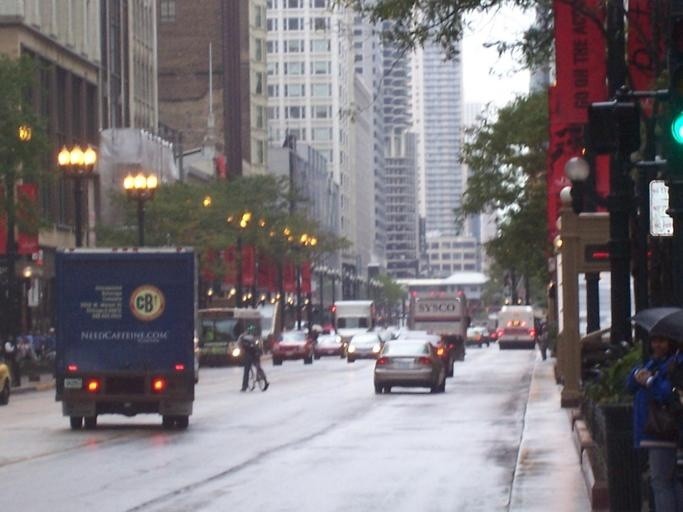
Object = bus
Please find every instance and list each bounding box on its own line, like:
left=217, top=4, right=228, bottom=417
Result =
left=493, top=303, right=537, bottom=351
left=197, top=305, right=264, bottom=367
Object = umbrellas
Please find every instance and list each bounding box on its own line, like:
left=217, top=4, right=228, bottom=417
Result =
left=631, top=304, right=682, bottom=350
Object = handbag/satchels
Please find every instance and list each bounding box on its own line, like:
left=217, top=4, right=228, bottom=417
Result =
left=645, top=397, right=682, bottom=441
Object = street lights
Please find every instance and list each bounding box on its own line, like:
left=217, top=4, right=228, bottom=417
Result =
left=54, top=139, right=100, bottom=248
left=7, top=123, right=34, bottom=389
left=120, top=171, right=158, bottom=249
left=548, top=157, right=600, bottom=385
left=200, top=194, right=319, bottom=330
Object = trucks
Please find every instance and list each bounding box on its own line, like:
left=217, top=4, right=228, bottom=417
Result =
left=53, top=243, right=198, bottom=432
left=330, top=298, right=377, bottom=337
left=403, top=282, right=470, bottom=348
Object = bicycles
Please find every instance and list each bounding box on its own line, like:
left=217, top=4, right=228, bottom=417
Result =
left=236, top=349, right=269, bottom=394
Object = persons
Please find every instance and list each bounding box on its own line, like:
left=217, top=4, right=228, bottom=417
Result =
left=624, top=334, right=682, bottom=511
left=1, top=326, right=56, bottom=365
left=535, top=321, right=548, bottom=361
left=231, top=324, right=269, bottom=392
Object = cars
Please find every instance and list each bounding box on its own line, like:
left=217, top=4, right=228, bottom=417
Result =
left=372, top=339, right=448, bottom=396
left=0, top=360, right=12, bottom=407
left=268, top=320, right=495, bottom=369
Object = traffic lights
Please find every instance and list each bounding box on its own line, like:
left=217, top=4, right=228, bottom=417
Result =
left=655, top=1, right=683, bottom=166
